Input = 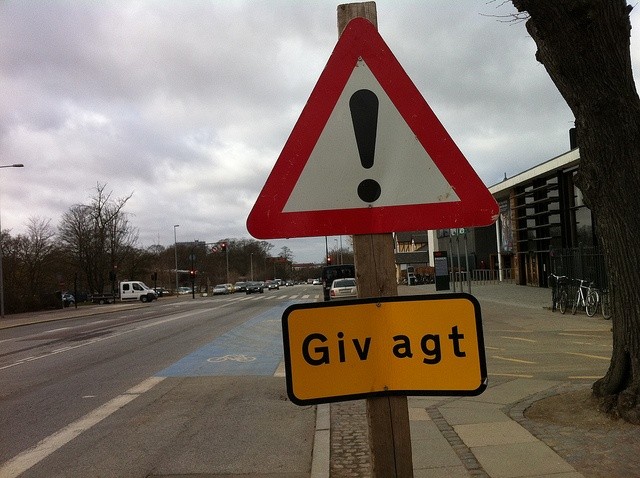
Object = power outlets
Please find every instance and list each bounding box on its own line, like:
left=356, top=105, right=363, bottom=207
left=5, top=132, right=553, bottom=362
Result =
left=89, top=281, right=155, bottom=304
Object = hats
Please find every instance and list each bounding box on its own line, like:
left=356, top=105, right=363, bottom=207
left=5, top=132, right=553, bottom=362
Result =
left=329, top=277, right=356, bottom=300
left=61, top=293, right=76, bottom=308
left=268, top=281, right=279, bottom=290
left=173, top=287, right=193, bottom=295
left=225, top=284, right=235, bottom=294
left=246, top=282, right=263, bottom=294
left=212, top=283, right=229, bottom=295
left=286, top=281, right=294, bottom=287
left=239, top=282, right=253, bottom=292
left=312, top=280, right=321, bottom=285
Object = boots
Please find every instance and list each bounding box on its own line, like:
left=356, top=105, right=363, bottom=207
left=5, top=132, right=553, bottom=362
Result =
left=152, top=287, right=170, bottom=294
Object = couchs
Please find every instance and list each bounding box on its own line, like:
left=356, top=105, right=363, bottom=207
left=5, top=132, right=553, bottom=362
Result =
left=327, top=256, right=332, bottom=265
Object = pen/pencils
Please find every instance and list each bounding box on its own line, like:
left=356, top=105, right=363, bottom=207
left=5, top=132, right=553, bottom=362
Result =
left=321, top=264, right=355, bottom=301
